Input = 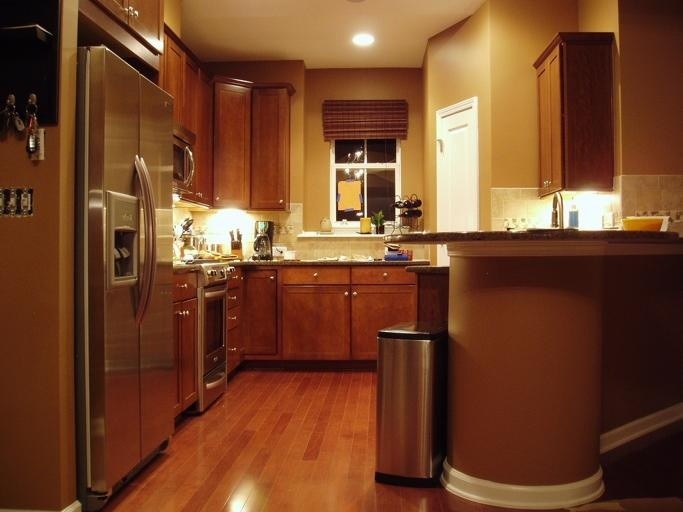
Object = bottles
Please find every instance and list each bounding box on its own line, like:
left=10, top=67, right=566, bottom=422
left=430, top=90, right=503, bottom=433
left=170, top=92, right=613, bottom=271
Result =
left=392, top=199, right=422, bottom=218
left=504, top=218, right=528, bottom=231
left=569, top=197, right=579, bottom=227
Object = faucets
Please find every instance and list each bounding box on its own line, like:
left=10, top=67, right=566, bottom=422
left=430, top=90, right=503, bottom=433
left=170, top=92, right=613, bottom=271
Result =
left=551, top=193, right=563, bottom=229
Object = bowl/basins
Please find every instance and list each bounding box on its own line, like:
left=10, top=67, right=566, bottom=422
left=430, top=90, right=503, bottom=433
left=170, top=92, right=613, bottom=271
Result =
left=621, top=218, right=664, bottom=231
left=626, top=216, right=670, bottom=232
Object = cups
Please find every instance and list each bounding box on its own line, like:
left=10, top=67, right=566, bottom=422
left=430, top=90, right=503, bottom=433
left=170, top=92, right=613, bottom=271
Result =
left=401, top=226, right=409, bottom=234
left=284, top=251, right=296, bottom=260
left=360, top=217, right=372, bottom=234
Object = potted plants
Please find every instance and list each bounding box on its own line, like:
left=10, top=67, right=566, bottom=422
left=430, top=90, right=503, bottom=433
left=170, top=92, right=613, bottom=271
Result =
left=371, top=210, right=387, bottom=234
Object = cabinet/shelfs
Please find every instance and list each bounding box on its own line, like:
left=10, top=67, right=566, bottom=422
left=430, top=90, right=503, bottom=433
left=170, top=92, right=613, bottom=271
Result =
left=78, top=1, right=164, bottom=76
left=533, top=31, right=614, bottom=200
left=214, top=78, right=292, bottom=212
left=173, top=266, right=417, bottom=426
left=159, top=27, right=214, bottom=208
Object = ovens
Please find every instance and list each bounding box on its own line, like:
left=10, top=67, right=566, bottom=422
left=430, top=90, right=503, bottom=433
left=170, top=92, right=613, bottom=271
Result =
left=198, top=286, right=229, bottom=413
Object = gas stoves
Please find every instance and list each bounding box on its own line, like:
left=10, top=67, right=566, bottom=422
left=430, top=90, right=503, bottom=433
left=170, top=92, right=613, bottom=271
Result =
left=174, top=261, right=236, bottom=286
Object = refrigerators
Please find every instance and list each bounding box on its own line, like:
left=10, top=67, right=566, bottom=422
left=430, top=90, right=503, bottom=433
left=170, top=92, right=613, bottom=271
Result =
left=76, top=46, right=174, bottom=509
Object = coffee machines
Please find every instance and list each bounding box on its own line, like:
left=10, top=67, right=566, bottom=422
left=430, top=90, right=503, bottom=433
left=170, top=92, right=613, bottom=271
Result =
left=252, top=220, right=274, bottom=262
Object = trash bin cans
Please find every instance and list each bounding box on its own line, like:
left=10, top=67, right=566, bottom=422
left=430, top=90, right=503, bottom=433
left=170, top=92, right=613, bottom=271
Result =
left=374, top=324, right=448, bottom=488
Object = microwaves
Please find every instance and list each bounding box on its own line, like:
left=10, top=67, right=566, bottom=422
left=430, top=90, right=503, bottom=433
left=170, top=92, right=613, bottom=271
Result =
left=173, top=135, right=194, bottom=194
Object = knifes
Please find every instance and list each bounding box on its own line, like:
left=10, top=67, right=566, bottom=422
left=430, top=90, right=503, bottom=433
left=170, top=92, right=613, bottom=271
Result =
left=230, top=228, right=242, bottom=241
